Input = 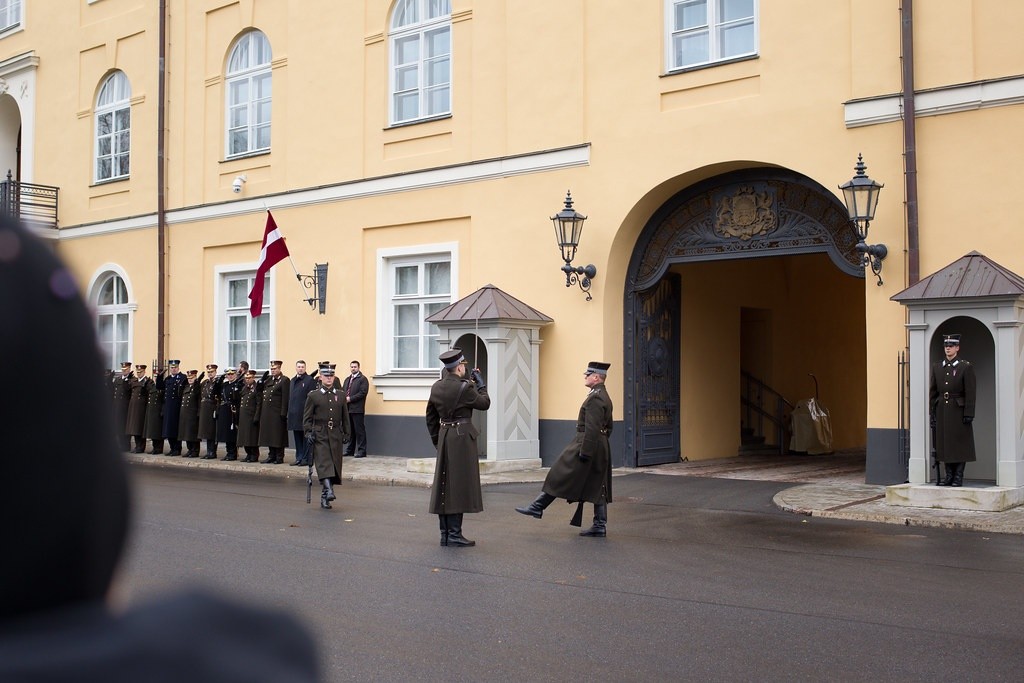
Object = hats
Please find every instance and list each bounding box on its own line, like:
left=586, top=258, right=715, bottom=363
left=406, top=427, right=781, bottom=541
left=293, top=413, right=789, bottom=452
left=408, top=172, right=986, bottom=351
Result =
left=439, top=349, right=468, bottom=368
left=584, top=362, right=611, bottom=374
left=943, top=334, right=962, bottom=346
left=319, top=364, right=336, bottom=375
left=121, top=361, right=283, bottom=377
left=318, top=361, right=329, bottom=365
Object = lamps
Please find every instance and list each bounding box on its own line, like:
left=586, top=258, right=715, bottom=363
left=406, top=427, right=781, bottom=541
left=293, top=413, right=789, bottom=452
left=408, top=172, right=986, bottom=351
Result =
left=838, top=151, right=888, bottom=286
left=549, top=188, right=596, bottom=302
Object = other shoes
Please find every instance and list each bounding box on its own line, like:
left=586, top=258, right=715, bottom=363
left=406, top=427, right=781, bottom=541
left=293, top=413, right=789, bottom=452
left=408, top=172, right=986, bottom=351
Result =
left=354, top=453, right=362, bottom=458
left=342, top=451, right=349, bottom=456
left=290, top=461, right=301, bottom=466
left=298, top=461, right=308, bottom=466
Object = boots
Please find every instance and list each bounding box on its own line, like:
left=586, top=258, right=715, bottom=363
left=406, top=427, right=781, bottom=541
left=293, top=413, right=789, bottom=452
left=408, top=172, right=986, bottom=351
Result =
left=940, top=462, right=966, bottom=487
left=439, top=514, right=447, bottom=547
left=120, top=435, right=283, bottom=464
left=514, top=492, right=555, bottom=518
left=445, top=513, right=476, bottom=547
left=320, top=487, right=332, bottom=509
left=580, top=500, right=607, bottom=537
left=322, top=478, right=336, bottom=501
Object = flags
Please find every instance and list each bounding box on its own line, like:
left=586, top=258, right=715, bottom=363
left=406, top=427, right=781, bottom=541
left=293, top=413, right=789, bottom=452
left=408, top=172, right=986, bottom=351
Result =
left=249, top=210, right=290, bottom=318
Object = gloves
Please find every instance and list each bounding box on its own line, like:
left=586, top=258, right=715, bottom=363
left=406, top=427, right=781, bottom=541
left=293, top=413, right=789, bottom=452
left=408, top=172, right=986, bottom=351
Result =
left=343, top=438, right=349, bottom=444
left=310, top=369, right=318, bottom=378
left=471, top=369, right=486, bottom=393
left=963, top=416, right=973, bottom=424
left=109, top=369, right=269, bottom=382
left=307, top=435, right=315, bottom=446
left=579, top=454, right=588, bottom=462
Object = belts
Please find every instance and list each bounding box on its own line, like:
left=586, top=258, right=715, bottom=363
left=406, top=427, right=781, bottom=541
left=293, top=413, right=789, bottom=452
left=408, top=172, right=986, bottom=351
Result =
left=939, top=392, right=961, bottom=399
left=441, top=418, right=471, bottom=425
left=576, top=426, right=606, bottom=433
left=316, top=421, right=339, bottom=429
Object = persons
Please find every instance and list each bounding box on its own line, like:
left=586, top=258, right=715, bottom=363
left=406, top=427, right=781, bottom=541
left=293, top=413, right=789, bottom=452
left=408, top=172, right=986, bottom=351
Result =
left=305, top=364, right=351, bottom=508
left=514, top=362, right=613, bottom=537
left=426, top=347, right=484, bottom=546
left=929, top=333, right=977, bottom=486
left=107, top=360, right=369, bottom=466
